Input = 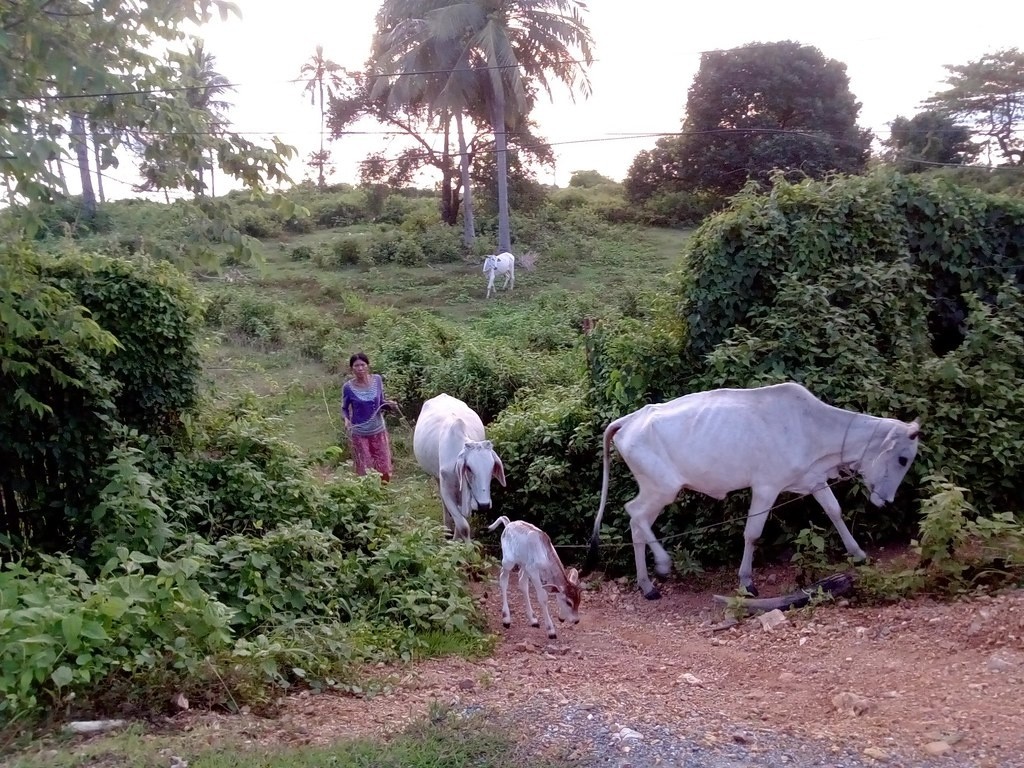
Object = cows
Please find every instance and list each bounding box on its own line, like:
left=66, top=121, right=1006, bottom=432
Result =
left=482, top=252, right=514, bottom=297
left=413, top=382, right=923, bottom=639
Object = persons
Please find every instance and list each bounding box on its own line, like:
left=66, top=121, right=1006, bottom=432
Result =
left=341, top=352, right=399, bottom=490
